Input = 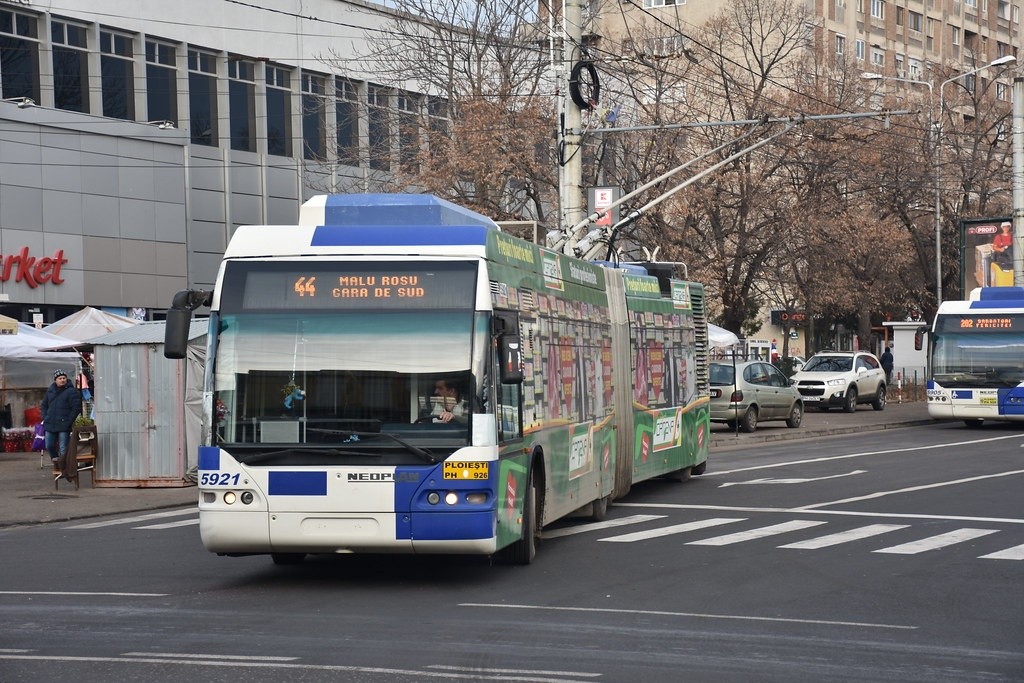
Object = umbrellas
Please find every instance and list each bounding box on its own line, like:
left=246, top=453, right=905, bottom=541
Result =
left=37, top=305, right=140, bottom=372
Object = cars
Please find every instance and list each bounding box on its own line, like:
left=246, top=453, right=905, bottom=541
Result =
left=708, top=361, right=804, bottom=433
left=778, top=355, right=807, bottom=372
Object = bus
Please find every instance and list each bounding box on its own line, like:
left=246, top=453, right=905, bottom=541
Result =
left=164, top=113, right=805, bottom=565
left=915, top=286, right=1024, bottom=429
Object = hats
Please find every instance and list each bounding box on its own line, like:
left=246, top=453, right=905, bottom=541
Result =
left=54, top=369, right=67, bottom=381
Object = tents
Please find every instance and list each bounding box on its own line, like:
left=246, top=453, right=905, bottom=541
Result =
left=1, top=314, right=83, bottom=412
left=708, top=323, right=740, bottom=438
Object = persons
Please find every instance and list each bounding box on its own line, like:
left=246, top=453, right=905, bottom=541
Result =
left=993, top=221, right=1013, bottom=270
left=879, top=346, right=893, bottom=385
left=415, top=374, right=470, bottom=427
left=536, top=300, right=695, bottom=423
left=40, top=368, right=83, bottom=479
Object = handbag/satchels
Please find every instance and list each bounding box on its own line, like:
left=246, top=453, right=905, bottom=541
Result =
left=31, top=420, right=46, bottom=449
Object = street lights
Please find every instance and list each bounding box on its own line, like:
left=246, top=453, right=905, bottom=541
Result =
left=860, top=54, right=1017, bottom=308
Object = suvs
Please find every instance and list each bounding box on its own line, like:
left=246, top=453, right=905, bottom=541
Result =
left=789, top=350, right=887, bottom=413
